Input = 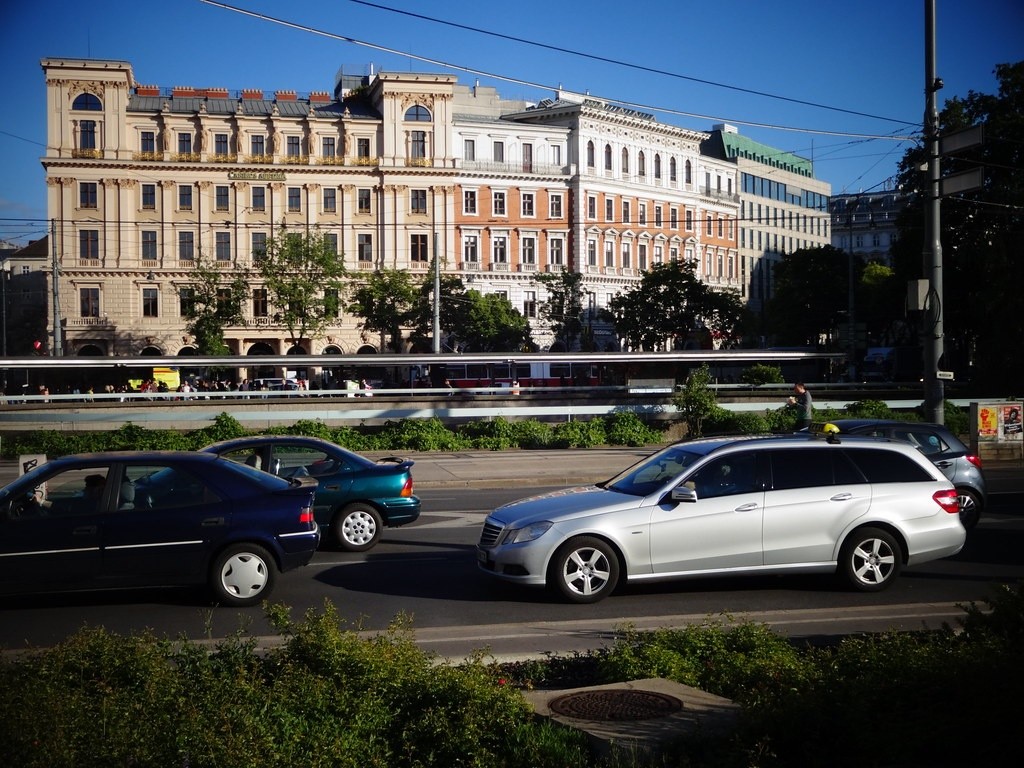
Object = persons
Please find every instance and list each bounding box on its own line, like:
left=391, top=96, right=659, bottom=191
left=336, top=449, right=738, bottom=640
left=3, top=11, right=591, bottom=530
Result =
left=0, top=366, right=459, bottom=406
left=1004, top=409, right=1022, bottom=435
left=28, top=474, right=107, bottom=518
left=787, top=381, right=814, bottom=432
left=683, top=462, right=745, bottom=500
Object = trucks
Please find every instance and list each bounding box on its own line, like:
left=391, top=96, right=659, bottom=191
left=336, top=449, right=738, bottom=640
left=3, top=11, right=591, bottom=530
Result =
left=858, top=346, right=923, bottom=381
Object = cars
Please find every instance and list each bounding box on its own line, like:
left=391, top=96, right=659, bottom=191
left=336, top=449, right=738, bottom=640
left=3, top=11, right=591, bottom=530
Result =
left=127, top=434, right=421, bottom=552
left=222, top=378, right=298, bottom=399
left=0, top=450, right=321, bottom=607
left=318, top=380, right=373, bottom=398
left=245, top=383, right=302, bottom=399
left=793, top=420, right=987, bottom=531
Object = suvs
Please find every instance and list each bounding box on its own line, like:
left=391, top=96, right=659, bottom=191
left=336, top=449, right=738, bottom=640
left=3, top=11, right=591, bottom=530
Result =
left=474, top=422, right=967, bottom=604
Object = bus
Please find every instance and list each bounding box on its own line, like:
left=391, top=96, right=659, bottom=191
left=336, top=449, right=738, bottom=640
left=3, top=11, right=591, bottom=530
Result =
left=405, top=364, right=599, bottom=395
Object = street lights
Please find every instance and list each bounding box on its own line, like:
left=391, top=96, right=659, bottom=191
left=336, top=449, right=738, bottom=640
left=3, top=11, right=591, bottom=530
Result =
left=586, top=290, right=596, bottom=318
left=849, top=202, right=877, bottom=349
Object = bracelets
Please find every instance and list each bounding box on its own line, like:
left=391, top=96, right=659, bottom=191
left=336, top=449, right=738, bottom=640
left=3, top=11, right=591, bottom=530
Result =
left=40, top=500, right=46, bottom=506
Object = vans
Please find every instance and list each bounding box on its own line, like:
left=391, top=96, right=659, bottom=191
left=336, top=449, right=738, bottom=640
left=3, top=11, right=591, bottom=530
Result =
left=733, top=347, right=825, bottom=383
